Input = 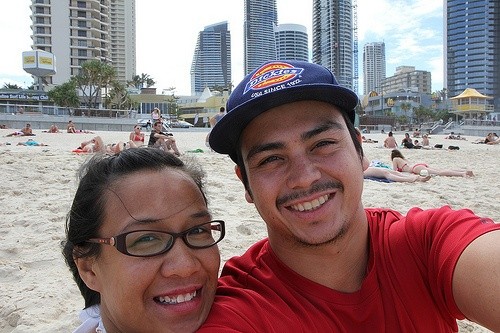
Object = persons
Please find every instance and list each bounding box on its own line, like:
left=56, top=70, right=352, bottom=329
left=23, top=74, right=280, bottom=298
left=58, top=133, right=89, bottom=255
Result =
left=364, top=166, right=431, bottom=182
left=62, top=147, right=225, bottom=333
left=391, top=149, right=474, bottom=177
left=189, top=60, right=500, bottom=333
left=6, top=124, right=32, bottom=137
left=485, top=132, right=500, bottom=144
left=78, top=125, right=145, bottom=153
left=215, top=107, right=226, bottom=122
left=362, top=126, right=468, bottom=149
left=47, top=124, right=62, bottom=133
left=152, top=107, right=162, bottom=122
left=0, top=124, right=6, bottom=129
left=18, top=106, right=24, bottom=114
left=148, top=122, right=180, bottom=156
left=68, top=121, right=76, bottom=133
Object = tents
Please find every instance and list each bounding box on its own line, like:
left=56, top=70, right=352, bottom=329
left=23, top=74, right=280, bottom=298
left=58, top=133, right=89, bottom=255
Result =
left=453, top=88, right=493, bottom=104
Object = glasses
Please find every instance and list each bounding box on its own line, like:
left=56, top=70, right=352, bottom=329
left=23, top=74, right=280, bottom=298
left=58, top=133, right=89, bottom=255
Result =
left=82, top=220, right=225, bottom=257
left=135, top=128, right=142, bottom=130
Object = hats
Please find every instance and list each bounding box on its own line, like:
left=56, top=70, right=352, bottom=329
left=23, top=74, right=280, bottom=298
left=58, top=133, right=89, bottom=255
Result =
left=208, top=60, right=358, bottom=154
